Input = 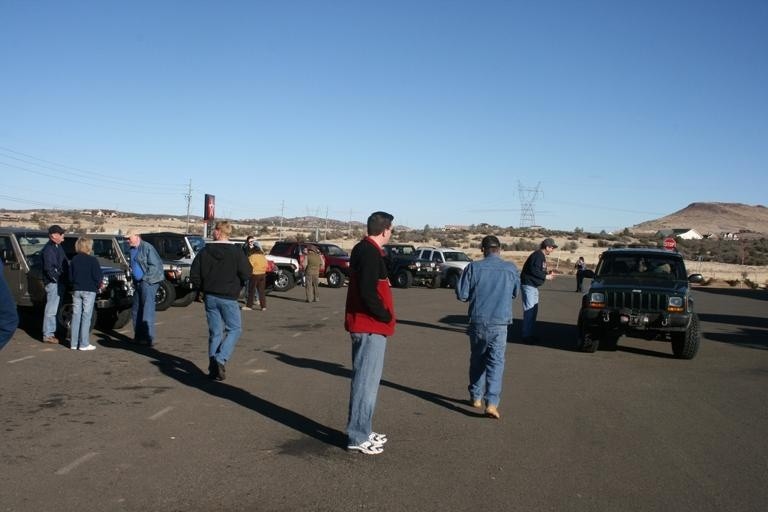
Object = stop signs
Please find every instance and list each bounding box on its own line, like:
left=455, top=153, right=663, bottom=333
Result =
left=663, top=237, right=677, bottom=251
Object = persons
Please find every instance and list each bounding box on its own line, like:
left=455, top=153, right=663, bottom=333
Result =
left=38, top=225, right=69, bottom=343
left=124, top=228, right=166, bottom=347
left=241, top=247, right=268, bottom=311
left=518, top=239, right=558, bottom=342
left=455, top=236, right=524, bottom=415
left=243, top=236, right=260, bottom=303
left=344, top=211, right=395, bottom=455
left=302, top=245, right=319, bottom=301
left=574, top=257, right=585, bottom=293
left=299, top=247, right=308, bottom=288
left=67, top=237, right=102, bottom=351
left=190, top=219, right=253, bottom=381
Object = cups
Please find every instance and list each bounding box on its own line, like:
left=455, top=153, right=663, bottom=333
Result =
left=549, top=270, right=554, bottom=276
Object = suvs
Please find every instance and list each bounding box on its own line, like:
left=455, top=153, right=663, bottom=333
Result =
left=0, top=226, right=136, bottom=340
left=574, top=243, right=702, bottom=360
left=268, top=240, right=352, bottom=289
left=206, top=236, right=301, bottom=292
left=137, top=232, right=209, bottom=311
left=415, top=245, right=476, bottom=288
left=64, top=234, right=192, bottom=312
left=380, top=243, right=442, bottom=289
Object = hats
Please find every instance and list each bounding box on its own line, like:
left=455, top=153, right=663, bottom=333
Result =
left=48, top=224, right=64, bottom=233
left=123, top=228, right=140, bottom=239
left=481, top=235, right=500, bottom=251
left=542, top=238, right=558, bottom=249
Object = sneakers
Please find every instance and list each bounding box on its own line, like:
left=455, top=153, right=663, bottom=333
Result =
left=346, top=441, right=385, bottom=455
left=208, top=360, right=227, bottom=379
left=367, top=431, right=388, bottom=446
left=241, top=295, right=266, bottom=310
left=484, top=404, right=499, bottom=419
left=42, top=334, right=97, bottom=351
left=470, top=399, right=482, bottom=407
